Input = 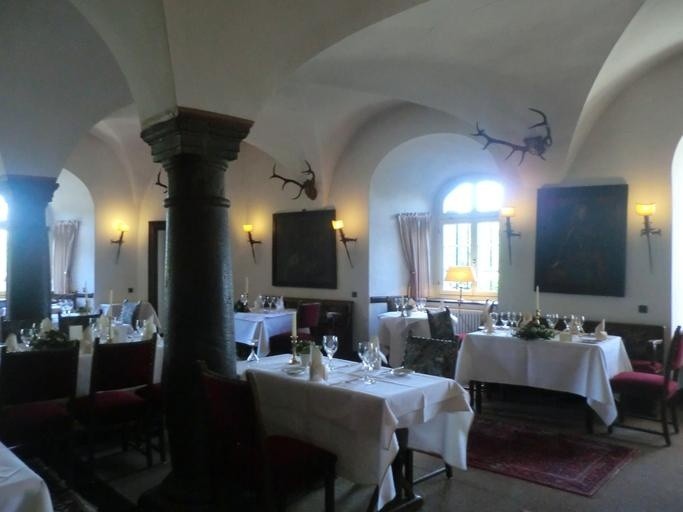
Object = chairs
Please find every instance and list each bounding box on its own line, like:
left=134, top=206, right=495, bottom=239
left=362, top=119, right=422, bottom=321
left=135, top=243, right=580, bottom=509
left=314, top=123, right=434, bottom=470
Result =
left=67, top=332, right=157, bottom=477
left=396, top=332, right=454, bottom=499
left=1, top=294, right=474, bottom=400
left=130, top=384, right=164, bottom=459
left=607, top=325, right=683, bottom=446
left=201, top=362, right=339, bottom=511
left=1, top=340, right=80, bottom=480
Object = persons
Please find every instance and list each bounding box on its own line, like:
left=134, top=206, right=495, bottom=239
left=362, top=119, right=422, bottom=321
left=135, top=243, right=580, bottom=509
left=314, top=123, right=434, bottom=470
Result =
left=550, top=200, right=615, bottom=288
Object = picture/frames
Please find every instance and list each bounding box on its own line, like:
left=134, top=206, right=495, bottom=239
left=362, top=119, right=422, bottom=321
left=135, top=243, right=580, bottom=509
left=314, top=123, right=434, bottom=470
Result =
left=272, top=209, right=337, bottom=289
left=534, top=184, right=627, bottom=297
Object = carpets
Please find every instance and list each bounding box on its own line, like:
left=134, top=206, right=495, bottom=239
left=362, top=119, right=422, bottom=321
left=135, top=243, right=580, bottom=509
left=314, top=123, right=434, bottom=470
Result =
left=465, top=407, right=642, bottom=498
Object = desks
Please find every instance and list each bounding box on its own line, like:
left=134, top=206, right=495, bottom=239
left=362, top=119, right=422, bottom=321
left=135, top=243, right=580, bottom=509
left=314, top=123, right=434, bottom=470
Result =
left=454, top=325, right=635, bottom=433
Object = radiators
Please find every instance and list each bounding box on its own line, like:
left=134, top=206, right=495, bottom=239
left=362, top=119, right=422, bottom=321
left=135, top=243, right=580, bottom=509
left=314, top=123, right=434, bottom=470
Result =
left=448, top=309, right=484, bottom=337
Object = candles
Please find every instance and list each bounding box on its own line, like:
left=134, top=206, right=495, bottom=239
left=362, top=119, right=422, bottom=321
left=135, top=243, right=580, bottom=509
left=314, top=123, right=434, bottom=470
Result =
left=110, top=289, right=112, bottom=305
left=245, top=276, right=249, bottom=293
left=535, top=285, right=539, bottom=310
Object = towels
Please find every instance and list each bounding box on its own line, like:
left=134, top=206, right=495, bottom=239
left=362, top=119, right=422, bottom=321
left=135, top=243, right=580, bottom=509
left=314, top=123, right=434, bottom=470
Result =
left=487, top=312, right=495, bottom=334
left=595, top=319, right=608, bottom=341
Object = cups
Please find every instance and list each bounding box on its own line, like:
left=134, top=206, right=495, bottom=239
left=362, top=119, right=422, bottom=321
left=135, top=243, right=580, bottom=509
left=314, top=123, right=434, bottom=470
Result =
left=21, top=317, right=146, bottom=347
left=395, top=297, right=426, bottom=313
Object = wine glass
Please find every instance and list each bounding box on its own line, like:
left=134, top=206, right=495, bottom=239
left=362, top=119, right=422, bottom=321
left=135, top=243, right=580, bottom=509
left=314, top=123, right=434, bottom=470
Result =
left=323, top=336, right=339, bottom=370
left=58, top=300, right=73, bottom=316
left=240, top=291, right=282, bottom=311
left=358, top=341, right=380, bottom=385
left=490, top=312, right=585, bottom=335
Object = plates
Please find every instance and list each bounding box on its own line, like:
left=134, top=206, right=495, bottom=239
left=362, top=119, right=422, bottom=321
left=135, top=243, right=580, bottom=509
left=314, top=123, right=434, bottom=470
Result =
left=390, top=370, right=413, bottom=377
left=282, top=367, right=305, bottom=375
left=580, top=335, right=607, bottom=341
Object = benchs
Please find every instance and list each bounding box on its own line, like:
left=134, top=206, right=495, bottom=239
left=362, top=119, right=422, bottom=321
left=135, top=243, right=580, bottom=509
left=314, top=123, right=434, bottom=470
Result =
left=556, top=319, right=664, bottom=374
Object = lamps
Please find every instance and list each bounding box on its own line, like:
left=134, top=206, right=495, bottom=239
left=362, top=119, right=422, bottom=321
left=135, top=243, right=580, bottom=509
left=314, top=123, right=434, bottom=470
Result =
left=110, top=224, right=129, bottom=265
left=635, top=204, right=661, bottom=274
left=500, top=207, right=521, bottom=266
left=243, top=225, right=262, bottom=263
left=444, top=266, right=474, bottom=308
left=331, top=220, right=357, bottom=269
